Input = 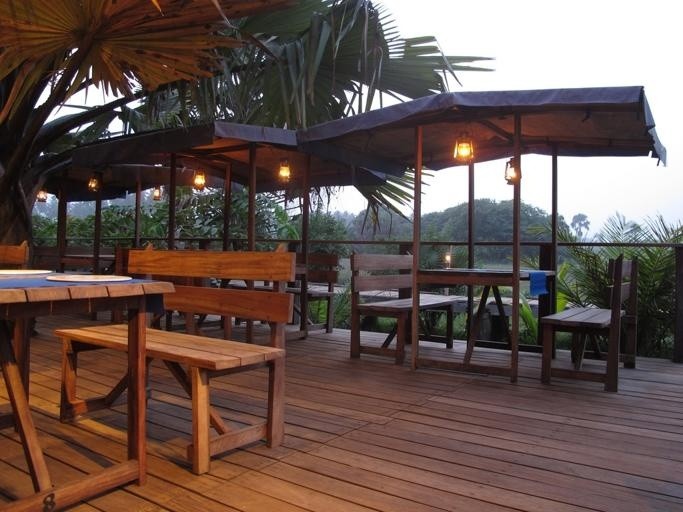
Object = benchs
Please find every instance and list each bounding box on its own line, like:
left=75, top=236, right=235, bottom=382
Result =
left=0, top=240, right=35, bottom=432
left=229, top=252, right=339, bottom=337
left=540, top=252, right=639, bottom=393
left=53, top=250, right=297, bottom=477
left=349, top=254, right=458, bottom=366
left=30, top=247, right=68, bottom=271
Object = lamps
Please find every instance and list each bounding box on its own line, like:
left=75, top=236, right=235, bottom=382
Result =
left=192, top=167, right=206, bottom=192
left=277, top=158, right=291, bottom=187
left=36, top=184, right=47, bottom=203
left=88, top=175, right=99, bottom=194
left=453, top=132, right=475, bottom=164
left=152, top=185, right=163, bottom=202
left=504, top=157, right=522, bottom=185
